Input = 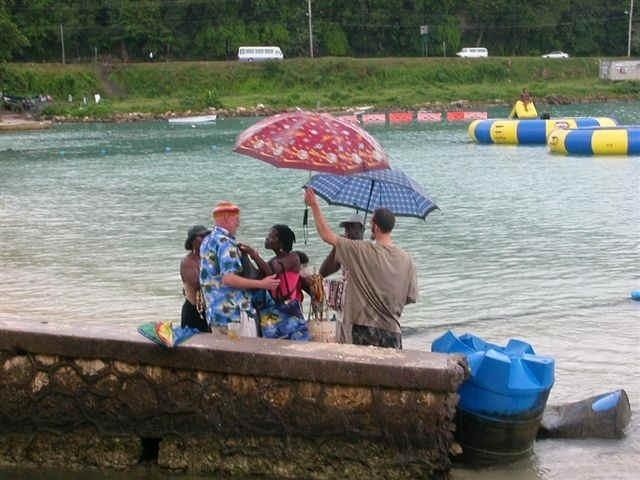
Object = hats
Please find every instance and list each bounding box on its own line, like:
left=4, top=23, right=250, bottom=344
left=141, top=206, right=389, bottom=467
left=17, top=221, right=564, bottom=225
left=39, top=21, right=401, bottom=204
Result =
left=183, top=224, right=212, bottom=251
left=338, top=214, right=367, bottom=228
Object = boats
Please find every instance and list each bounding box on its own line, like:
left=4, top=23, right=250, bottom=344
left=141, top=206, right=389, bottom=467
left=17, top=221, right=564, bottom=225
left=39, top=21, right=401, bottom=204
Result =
left=547, top=123, right=640, bottom=157
left=467, top=111, right=626, bottom=146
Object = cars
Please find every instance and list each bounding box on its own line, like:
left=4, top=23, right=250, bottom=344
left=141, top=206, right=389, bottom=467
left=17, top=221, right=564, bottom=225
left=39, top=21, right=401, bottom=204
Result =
left=541, top=50, right=569, bottom=59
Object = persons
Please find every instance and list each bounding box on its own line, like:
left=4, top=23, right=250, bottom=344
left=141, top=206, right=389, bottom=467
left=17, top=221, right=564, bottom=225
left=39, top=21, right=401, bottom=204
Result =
left=180, top=188, right=417, bottom=349
left=508, top=94, right=537, bottom=119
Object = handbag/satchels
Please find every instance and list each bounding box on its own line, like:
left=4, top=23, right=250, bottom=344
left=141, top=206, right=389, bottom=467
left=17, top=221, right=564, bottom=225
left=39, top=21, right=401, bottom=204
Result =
left=258, top=299, right=309, bottom=339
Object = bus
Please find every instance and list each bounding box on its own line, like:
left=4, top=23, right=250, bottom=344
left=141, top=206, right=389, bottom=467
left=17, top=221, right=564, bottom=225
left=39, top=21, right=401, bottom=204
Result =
left=236, top=46, right=285, bottom=62
left=455, top=47, right=489, bottom=58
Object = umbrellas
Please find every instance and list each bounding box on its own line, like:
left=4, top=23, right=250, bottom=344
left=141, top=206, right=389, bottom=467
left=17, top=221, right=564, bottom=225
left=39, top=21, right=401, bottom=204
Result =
left=233, top=111, right=442, bottom=233
